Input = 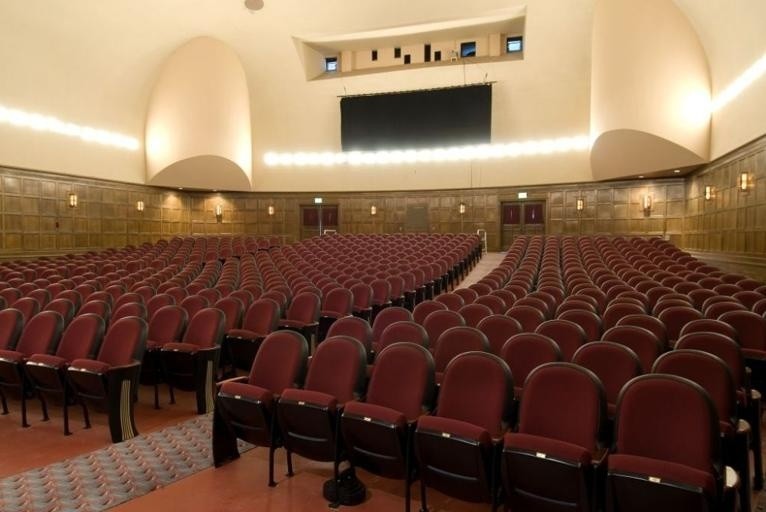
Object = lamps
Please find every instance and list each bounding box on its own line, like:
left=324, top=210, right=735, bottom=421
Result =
left=70, top=192, right=77, bottom=208
left=642, top=194, right=650, bottom=209
left=704, top=186, right=712, bottom=201
left=268, top=205, right=275, bottom=215
left=370, top=205, right=376, bottom=215
left=215, top=204, right=222, bottom=217
left=576, top=198, right=584, bottom=212
left=458, top=202, right=467, bottom=214
left=738, top=173, right=747, bottom=193
left=136, top=199, right=144, bottom=212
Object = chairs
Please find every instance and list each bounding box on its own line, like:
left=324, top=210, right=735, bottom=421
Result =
left=0, top=232, right=766, bottom=512
